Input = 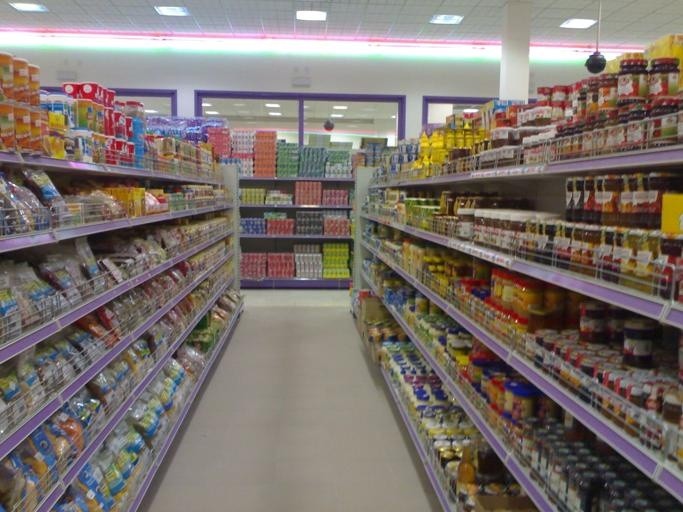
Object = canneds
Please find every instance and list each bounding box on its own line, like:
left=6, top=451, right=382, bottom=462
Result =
left=574, top=57, right=682, bottom=135
left=520, top=97, right=682, bottom=167
left=454, top=207, right=539, bottom=264
left=537, top=213, right=683, bottom=301
left=563, top=173, right=679, bottom=230
left=534, top=299, right=682, bottom=473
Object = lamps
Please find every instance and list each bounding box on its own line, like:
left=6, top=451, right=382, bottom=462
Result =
left=584, top=0, right=605, bottom=73
left=324, top=101, right=335, bottom=130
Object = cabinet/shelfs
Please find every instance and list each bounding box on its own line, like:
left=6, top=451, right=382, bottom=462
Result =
left=239, top=175, right=355, bottom=288
left=0, top=153, right=245, bottom=512
left=362, top=146, right=683, bottom=512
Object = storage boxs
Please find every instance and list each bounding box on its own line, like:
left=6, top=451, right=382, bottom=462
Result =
left=361, top=138, right=388, bottom=148
